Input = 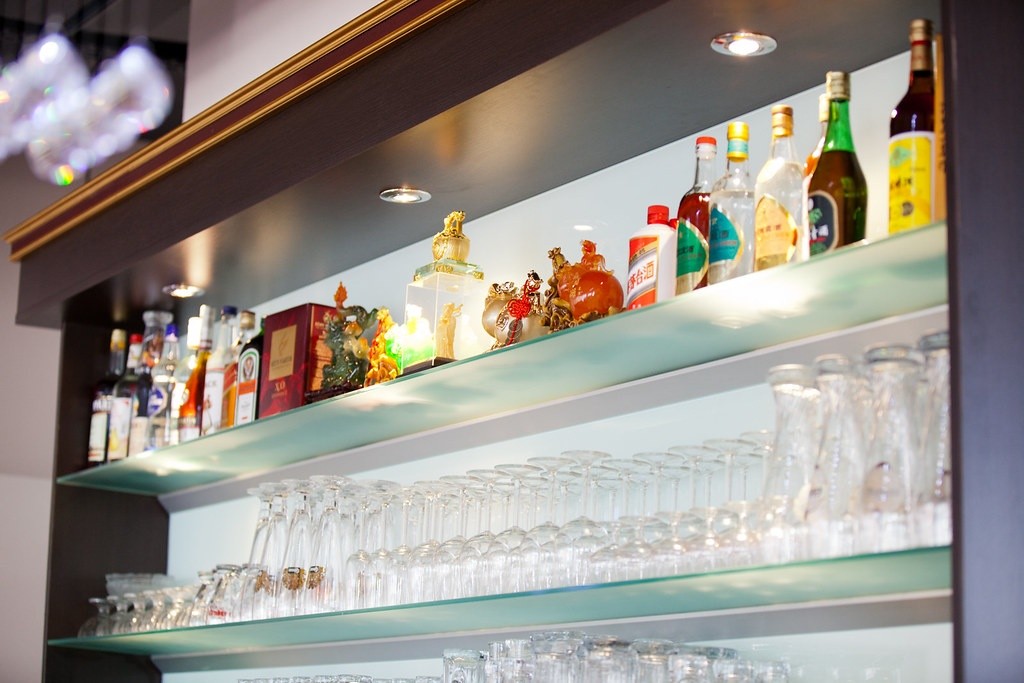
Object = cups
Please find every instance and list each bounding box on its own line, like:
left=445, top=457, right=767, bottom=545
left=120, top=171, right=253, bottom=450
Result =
left=756, top=327, right=951, bottom=566
left=134, top=311, right=174, bottom=376
left=236, top=629, right=901, bottom=683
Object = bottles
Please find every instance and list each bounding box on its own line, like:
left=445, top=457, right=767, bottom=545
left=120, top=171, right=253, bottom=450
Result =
left=200, top=304, right=239, bottom=437
left=170, top=316, right=202, bottom=445
left=87, top=329, right=127, bottom=465
left=234, top=315, right=270, bottom=426
left=105, top=333, right=143, bottom=463
left=220, top=309, right=257, bottom=427
left=889, top=16, right=934, bottom=238
left=809, top=69, right=867, bottom=256
left=626, top=204, right=675, bottom=311
left=143, top=322, right=181, bottom=450
left=675, top=135, right=718, bottom=299
left=127, top=327, right=161, bottom=458
left=754, top=104, right=805, bottom=271
left=799, top=93, right=831, bottom=261
left=706, top=121, right=756, bottom=284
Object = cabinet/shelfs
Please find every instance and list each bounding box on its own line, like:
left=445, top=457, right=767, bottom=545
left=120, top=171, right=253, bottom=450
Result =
left=4, top=0, right=1023, bottom=683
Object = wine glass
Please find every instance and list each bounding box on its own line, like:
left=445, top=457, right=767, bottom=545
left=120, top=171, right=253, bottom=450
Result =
left=88, top=429, right=776, bottom=635
